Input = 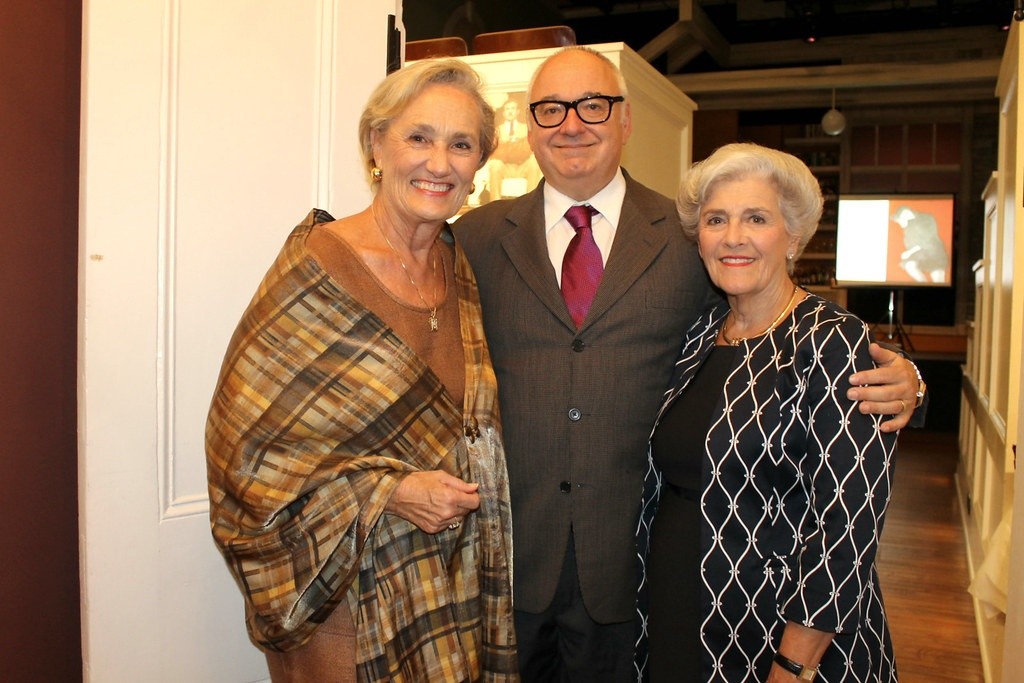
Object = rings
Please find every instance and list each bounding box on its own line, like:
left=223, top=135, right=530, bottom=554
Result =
left=447, top=516, right=460, bottom=529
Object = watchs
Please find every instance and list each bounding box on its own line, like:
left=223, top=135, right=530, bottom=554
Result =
left=774, top=651, right=817, bottom=682
left=905, top=358, right=926, bottom=408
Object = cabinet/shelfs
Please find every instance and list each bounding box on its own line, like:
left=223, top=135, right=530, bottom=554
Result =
left=952, top=10, right=1024, bottom=683
left=783, top=137, right=844, bottom=308
left=954, top=105, right=999, bottom=327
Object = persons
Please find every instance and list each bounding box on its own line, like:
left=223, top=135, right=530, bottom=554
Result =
left=448, top=48, right=926, bottom=683
left=628, top=143, right=898, bottom=683
left=205, top=56, right=522, bottom=683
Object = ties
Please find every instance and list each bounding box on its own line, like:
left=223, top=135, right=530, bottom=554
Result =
left=560, top=205, right=603, bottom=332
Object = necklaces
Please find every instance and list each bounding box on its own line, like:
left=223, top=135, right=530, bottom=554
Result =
left=722, top=285, right=799, bottom=346
left=372, top=204, right=439, bottom=331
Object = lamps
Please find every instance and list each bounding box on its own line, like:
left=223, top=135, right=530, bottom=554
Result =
left=822, top=89, right=845, bottom=136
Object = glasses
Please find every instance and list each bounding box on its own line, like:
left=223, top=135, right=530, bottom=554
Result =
left=530, top=94, right=625, bottom=129
